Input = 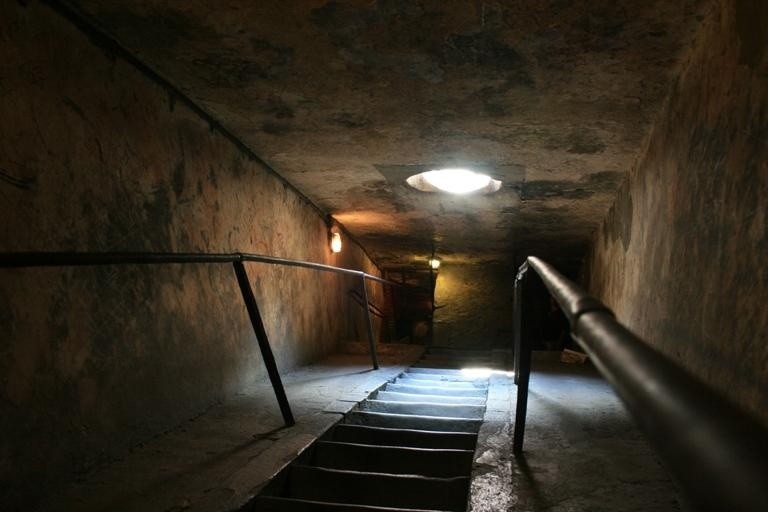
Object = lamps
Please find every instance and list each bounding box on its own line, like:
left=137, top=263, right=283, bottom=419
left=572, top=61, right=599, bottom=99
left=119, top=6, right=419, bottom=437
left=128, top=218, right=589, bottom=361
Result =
left=328, top=225, right=342, bottom=252
left=427, top=248, right=443, bottom=269
